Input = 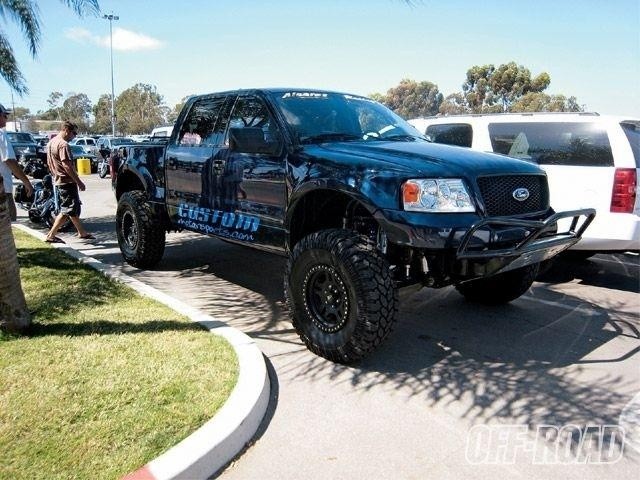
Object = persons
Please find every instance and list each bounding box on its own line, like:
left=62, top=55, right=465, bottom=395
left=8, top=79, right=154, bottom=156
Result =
left=44, top=121, right=97, bottom=247
left=0, top=104, right=34, bottom=221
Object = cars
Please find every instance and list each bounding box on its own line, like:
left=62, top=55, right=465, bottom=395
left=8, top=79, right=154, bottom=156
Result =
left=4, top=124, right=173, bottom=179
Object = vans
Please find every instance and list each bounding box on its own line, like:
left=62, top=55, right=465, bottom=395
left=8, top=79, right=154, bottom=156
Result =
left=376, top=112, right=640, bottom=267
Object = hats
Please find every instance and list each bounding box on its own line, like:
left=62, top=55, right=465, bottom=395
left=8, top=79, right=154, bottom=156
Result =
left=0, top=102, right=14, bottom=117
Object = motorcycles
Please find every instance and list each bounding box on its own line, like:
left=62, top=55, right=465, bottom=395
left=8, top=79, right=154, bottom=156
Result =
left=98, top=144, right=110, bottom=179
left=13, top=156, right=81, bottom=232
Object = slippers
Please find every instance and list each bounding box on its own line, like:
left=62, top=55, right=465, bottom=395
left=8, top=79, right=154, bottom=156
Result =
left=77, top=234, right=96, bottom=240
left=45, top=236, right=65, bottom=244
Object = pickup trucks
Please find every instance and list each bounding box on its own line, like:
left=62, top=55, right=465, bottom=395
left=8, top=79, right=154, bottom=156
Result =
left=109, top=88, right=596, bottom=366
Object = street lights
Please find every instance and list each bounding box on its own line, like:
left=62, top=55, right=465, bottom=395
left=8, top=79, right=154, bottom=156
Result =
left=101, top=12, right=120, bottom=137
